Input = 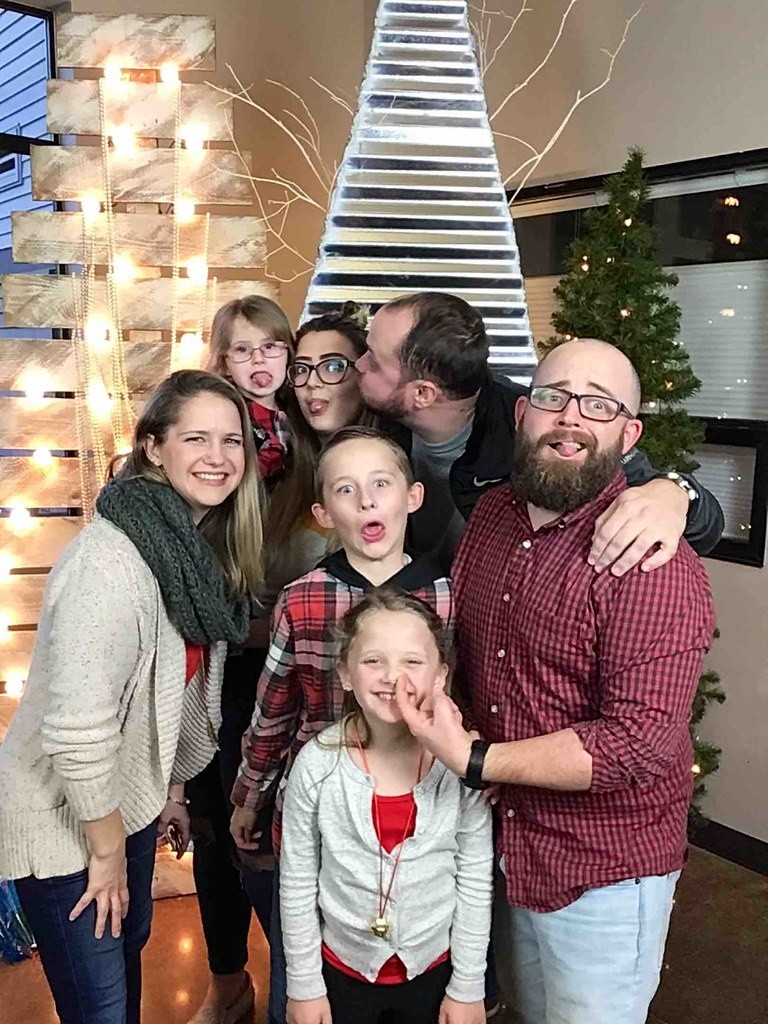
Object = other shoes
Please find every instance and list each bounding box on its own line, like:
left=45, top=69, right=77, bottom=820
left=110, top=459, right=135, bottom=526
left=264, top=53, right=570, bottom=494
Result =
left=187, top=972, right=257, bottom=1024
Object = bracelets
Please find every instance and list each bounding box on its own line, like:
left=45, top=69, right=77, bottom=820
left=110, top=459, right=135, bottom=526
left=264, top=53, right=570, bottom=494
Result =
left=167, top=791, right=190, bottom=806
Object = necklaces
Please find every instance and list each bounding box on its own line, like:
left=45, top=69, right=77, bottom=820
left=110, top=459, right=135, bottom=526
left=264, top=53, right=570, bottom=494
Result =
left=351, top=711, right=427, bottom=938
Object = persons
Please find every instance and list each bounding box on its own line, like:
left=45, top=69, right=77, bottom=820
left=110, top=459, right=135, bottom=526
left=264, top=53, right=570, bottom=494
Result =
left=1, top=289, right=726, bottom=1022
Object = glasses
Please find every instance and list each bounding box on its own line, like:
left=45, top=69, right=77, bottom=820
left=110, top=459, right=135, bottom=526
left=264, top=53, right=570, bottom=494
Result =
left=285, top=358, right=355, bottom=388
left=226, top=341, right=289, bottom=363
left=526, top=385, right=635, bottom=423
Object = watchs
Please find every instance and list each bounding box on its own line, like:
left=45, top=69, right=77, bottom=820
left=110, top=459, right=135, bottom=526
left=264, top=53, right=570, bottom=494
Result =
left=649, top=470, right=699, bottom=518
left=457, top=739, right=491, bottom=791
